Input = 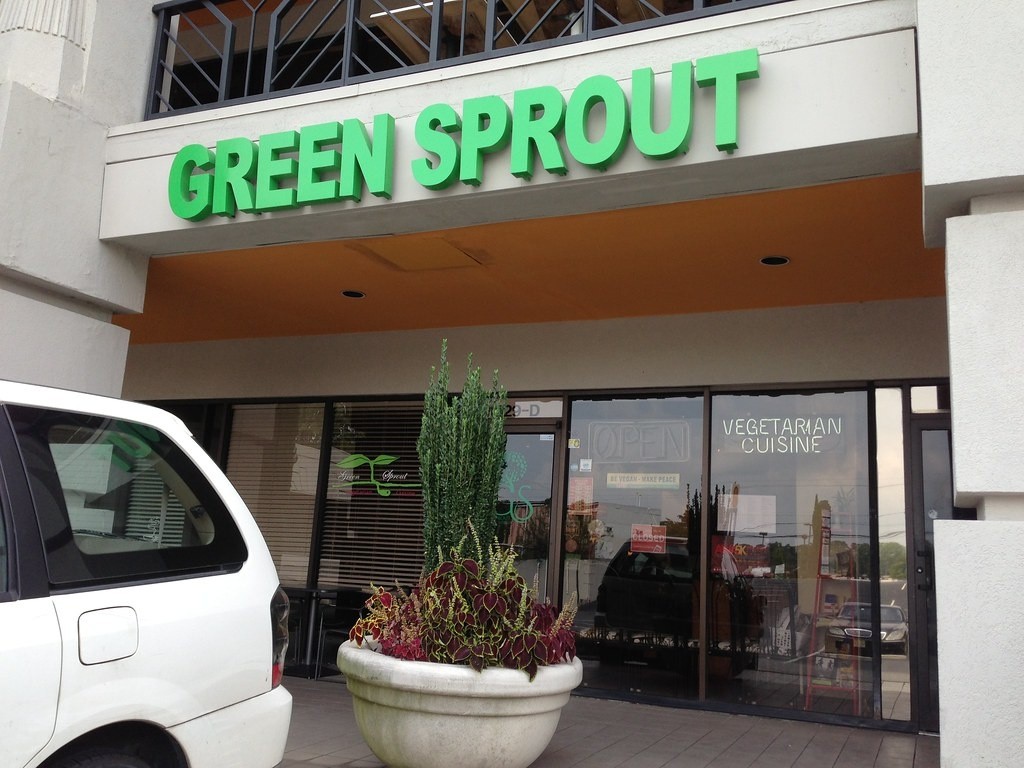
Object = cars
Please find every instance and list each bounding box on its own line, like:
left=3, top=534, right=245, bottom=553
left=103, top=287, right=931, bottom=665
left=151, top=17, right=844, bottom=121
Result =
left=824, top=599, right=909, bottom=657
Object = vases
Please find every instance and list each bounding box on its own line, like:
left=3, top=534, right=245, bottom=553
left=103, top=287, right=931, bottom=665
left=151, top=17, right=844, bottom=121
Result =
left=337, top=634, right=583, bottom=767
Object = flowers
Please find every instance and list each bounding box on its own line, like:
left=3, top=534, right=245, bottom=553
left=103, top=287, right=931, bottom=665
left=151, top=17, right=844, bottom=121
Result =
left=348, top=515, right=580, bottom=683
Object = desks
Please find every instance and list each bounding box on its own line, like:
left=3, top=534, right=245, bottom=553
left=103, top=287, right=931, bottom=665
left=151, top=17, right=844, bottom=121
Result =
left=279, top=590, right=344, bottom=679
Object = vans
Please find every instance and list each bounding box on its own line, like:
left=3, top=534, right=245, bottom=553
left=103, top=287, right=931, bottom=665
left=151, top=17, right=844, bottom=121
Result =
left=591, top=535, right=765, bottom=675
left=0, top=377, right=295, bottom=768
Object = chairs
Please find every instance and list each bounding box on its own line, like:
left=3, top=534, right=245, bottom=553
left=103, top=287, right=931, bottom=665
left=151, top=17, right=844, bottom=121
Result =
left=281, top=585, right=412, bottom=684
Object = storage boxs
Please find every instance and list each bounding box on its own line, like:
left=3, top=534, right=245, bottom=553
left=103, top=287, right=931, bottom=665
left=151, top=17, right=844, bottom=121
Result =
left=770, top=625, right=812, bottom=652
